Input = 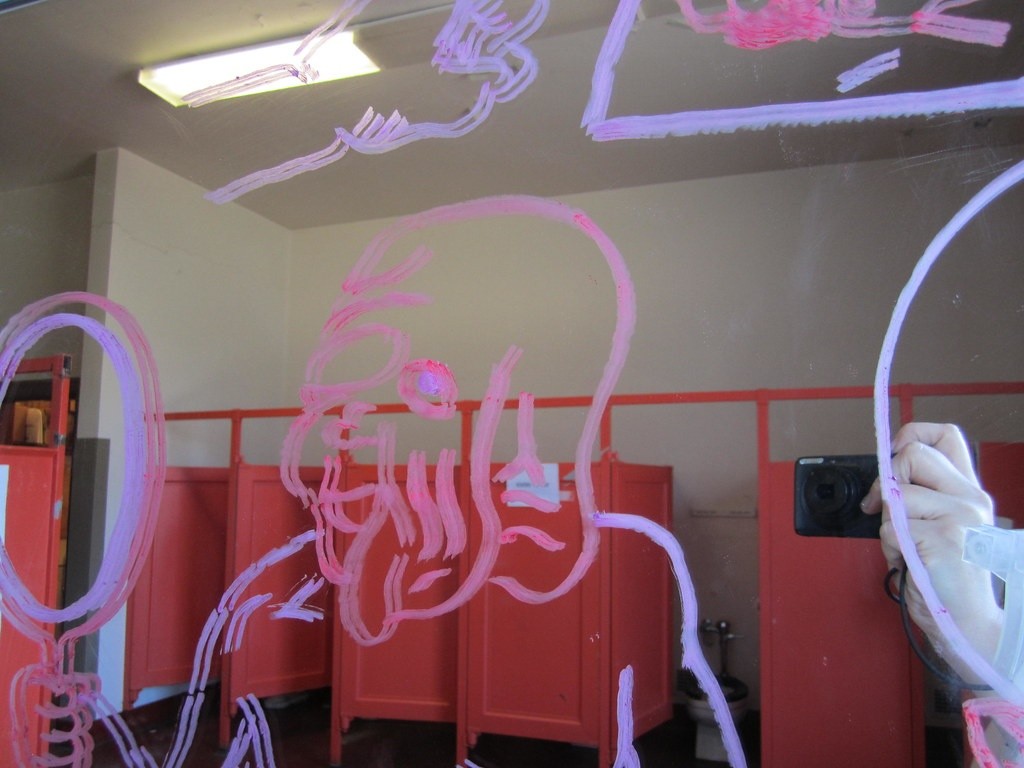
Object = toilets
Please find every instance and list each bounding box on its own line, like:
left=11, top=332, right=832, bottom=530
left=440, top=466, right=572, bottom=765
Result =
left=683, top=676, right=754, bottom=763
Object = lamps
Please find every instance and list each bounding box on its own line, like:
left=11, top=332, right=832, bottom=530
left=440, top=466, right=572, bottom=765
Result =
left=135, top=27, right=384, bottom=110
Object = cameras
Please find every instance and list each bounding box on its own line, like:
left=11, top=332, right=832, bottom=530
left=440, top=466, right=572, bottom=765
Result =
left=793, top=453, right=898, bottom=539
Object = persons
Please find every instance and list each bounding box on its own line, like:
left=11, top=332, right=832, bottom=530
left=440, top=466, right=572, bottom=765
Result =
left=859, top=421, right=1024, bottom=768
